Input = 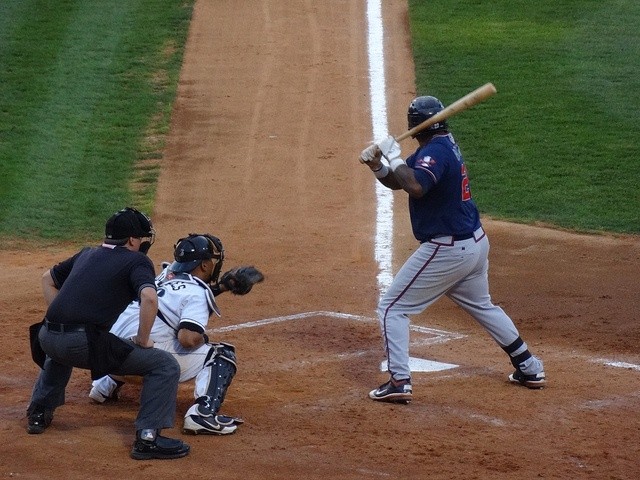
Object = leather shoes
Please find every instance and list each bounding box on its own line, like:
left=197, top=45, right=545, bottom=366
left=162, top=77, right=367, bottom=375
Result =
left=132, top=432, right=190, bottom=460
left=24, top=408, right=54, bottom=436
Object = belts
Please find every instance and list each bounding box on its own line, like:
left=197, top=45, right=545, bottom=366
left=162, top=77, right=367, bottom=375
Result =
left=39, top=317, right=92, bottom=334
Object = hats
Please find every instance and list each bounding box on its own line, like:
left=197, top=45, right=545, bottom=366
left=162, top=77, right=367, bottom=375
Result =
left=102, top=207, right=156, bottom=257
left=165, top=233, right=224, bottom=293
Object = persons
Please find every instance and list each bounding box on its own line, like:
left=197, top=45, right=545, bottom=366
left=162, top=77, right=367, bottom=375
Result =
left=88, top=233, right=266, bottom=436
left=360, top=95, right=547, bottom=403
left=25, top=206, right=191, bottom=461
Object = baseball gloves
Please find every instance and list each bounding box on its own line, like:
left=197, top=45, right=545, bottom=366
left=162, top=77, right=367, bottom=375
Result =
left=221, top=267, right=264, bottom=295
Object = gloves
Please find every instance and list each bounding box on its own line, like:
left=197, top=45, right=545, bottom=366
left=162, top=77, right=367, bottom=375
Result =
left=378, top=135, right=402, bottom=159
left=359, top=146, right=382, bottom=167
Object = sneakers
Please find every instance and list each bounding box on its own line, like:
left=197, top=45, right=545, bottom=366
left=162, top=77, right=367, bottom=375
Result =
left=182, top=411, right=238, bottom=436
left=87, top=382, right=120, bottom=404
left=367, top=378, right=415, bottom=405
left=508, top=367, right=548, bottom=391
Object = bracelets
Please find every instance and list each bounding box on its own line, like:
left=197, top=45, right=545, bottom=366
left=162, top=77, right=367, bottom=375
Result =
left=389, top=158, right=406, bottom=173
left=372, top=162, right=389, bottom=180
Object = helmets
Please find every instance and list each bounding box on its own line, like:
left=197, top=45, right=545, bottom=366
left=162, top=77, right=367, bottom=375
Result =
left=406, top=94, right=450, bottom=135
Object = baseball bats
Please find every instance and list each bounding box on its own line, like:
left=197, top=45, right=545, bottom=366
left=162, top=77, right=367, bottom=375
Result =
left=359, top=82, right=498, bottom=164
